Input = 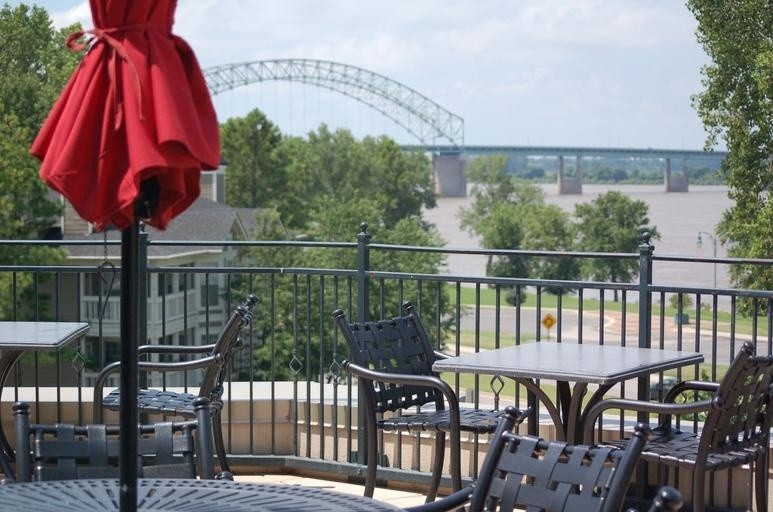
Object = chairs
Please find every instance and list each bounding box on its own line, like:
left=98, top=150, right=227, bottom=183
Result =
left=597, top=342, right=771, bottom=511
left=93, top=293, right=260, bottom=480
left=465, top=406, right=650, bottom=509
left=330, top=301, right=534, bottom=509
left=10, top=403, right=230, bottom=482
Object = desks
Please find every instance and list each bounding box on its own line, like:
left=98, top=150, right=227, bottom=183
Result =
left=0, top=476, right=401, bottom=510
left=0, top=320, right=89, bottom=475
left=433, top=341, right=705, bottom=486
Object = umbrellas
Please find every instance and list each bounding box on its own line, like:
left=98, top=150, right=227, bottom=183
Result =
left=29, top=0, right=221, bottom=511
